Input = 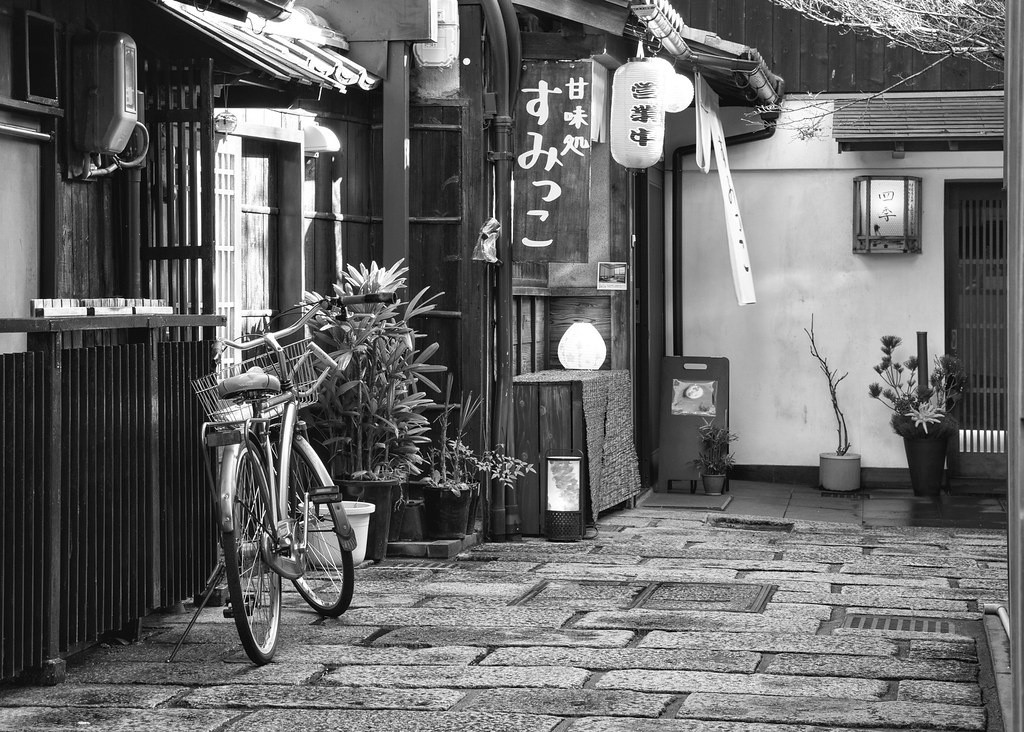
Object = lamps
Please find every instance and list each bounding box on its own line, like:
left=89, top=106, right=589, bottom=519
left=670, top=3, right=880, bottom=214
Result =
left=543, top=446, right=586, bottom=541
left=557, top=321, right=607, bottom=371
left=852, top=174, right=923, bottom=256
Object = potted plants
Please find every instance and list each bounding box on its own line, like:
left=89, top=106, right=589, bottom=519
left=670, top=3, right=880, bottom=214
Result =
left=804, top=313, right=861, bottom=492
left=686, top=413, right=739, bottom=497
left=868, top=332, right=968, bottom=498
left=299, top=256, right=536, bottom=561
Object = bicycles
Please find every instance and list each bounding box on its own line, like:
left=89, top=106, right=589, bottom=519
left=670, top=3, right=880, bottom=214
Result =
left=165, top=288, right=398, bottom=666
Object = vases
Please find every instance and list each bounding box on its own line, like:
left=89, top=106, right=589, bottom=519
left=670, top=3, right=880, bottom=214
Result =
left=297, top=501, right=376, bottom=565
